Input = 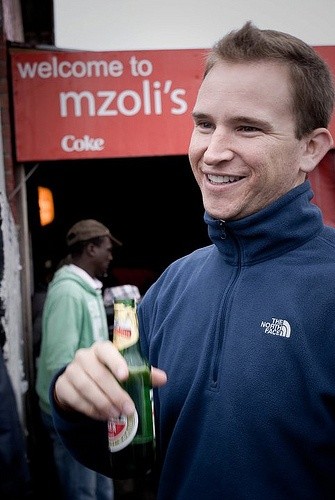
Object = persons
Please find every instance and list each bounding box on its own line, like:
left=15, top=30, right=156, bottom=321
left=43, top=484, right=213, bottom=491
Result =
left=50, top=22, right=335, bottom=498
left=34, top=221, right=121, bottom=500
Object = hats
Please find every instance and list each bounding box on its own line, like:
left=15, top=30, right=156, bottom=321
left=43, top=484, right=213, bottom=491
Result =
left=67, top=216, right=122, bottom=246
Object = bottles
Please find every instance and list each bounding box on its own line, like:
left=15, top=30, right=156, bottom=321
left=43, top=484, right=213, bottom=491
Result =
left=107, top=298, right=158, bottom=500
